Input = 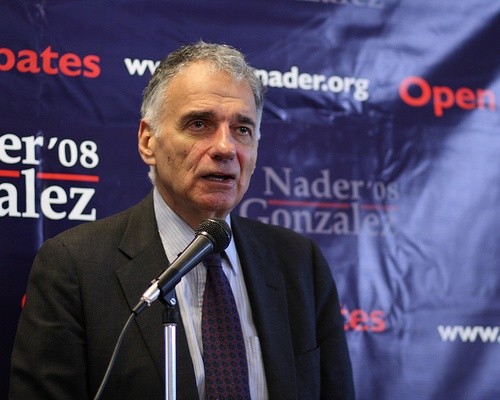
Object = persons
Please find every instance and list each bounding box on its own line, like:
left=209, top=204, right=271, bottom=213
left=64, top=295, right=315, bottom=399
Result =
left=10, top=41, right=356, bottom=400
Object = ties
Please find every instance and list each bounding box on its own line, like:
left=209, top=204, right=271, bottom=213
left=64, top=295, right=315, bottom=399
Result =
left=202, top=252, right=251, bottom=400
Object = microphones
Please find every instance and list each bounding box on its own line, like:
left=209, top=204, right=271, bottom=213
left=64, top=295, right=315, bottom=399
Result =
left=131, top=217, right=232, bottom=317
